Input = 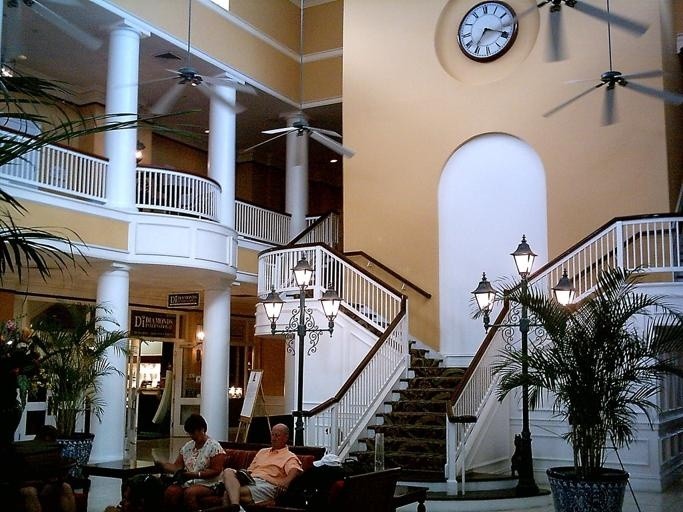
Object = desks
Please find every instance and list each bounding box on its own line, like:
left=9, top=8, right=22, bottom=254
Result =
left=394, top=480, right=429, bottom=511
left=83, top=457, right=175, bottom=512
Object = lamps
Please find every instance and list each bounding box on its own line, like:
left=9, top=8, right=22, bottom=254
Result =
left=194, top=323, right=204, bottom=350
left=136, top=139, right=145, bottom=163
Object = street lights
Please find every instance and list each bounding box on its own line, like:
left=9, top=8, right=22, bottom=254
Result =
left=468, top=230, right=576, bottom=494
left=257, top=251, right=344, bottom=448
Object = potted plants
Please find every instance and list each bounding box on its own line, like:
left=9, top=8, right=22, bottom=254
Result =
left=468, top=265, right=682, bottom=512
left=15, top=301, right=149, bottom=480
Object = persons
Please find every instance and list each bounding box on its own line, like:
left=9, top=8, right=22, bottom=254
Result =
left=222, top=424, right=304, bottom=512
left=16, top=425, right=76, bottom=512
left=153, top=413, right=226, bottom=512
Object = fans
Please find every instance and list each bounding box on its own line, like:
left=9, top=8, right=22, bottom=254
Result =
left=112, top=1, right=257, bottom=118
left=543, top=0, right=683, bottom=127
left=472, top=0, right=650, bottom=64
left=242, top=0, right=357, bottom=165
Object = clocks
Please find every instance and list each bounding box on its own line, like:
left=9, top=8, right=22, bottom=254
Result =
left=454, top=0, right=521, bottom=63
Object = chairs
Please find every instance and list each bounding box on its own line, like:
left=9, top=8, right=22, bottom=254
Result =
left=266, top=467, right=402, bottom=512
left=0, top=469, right=90, bottom=512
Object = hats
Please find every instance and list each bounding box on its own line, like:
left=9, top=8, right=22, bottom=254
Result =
left=312, top=454, right=342, bottom=468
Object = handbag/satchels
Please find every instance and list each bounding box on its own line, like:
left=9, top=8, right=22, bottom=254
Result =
left=341, top=458, right=367, bottom=476
left=127, top=472, right=164, bottom=501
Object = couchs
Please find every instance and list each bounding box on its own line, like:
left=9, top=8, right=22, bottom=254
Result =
left=127, top=440, right=325, bottom=512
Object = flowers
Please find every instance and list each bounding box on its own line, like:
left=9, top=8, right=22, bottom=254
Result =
left=0, top=319, right=44, bottom=410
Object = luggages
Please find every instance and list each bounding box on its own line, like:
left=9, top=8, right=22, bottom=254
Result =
left=282, top=467, right=331, bottom=508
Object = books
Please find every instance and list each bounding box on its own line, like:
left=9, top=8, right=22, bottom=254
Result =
left=234, top=468, right=256, bottom=485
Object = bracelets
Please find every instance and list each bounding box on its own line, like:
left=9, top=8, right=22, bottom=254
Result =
left=198, top=471, right=201, bottom=479
